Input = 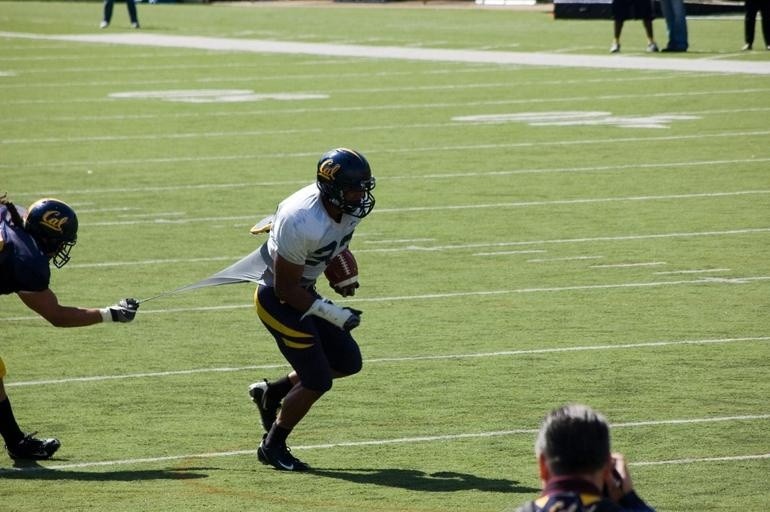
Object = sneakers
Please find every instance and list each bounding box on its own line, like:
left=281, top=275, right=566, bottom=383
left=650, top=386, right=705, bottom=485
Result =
left=610, top=42, right=658, bottom=53
left=246, top=377, right=278, bottom=433
left=741, top=44, right=752, bottom=50
left=99, top=20, right=140, bottom=29
left=256, top=433, right=311, bottom=471
left=4, top=429, right=61, bottom=461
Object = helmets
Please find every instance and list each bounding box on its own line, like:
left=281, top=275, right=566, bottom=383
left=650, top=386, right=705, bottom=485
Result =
left=21, top=197, right=79, bottom=269
left=315, top=147, right=378, bottom=219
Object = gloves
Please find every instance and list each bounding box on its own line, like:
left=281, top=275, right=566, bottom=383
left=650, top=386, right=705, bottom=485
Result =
left=341, top=305, right=363, bottom=333
left=109, top=297, right=140, bottom=323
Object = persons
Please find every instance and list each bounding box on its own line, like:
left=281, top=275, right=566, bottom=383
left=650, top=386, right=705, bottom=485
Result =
left=1, top=198, right=139, bottom=460
left=510, top=403, right=652, bottom=511
left=250, top=149, right=376, bottom=472
left=100, top=0, right=141, bottom=30
left=611, top=0, right=658, bottom=53
left=743, top=0, right=766, bottom=49
left=659, top=1, right=689, bottom=52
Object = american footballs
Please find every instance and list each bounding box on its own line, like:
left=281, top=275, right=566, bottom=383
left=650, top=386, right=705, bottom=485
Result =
left=324, top=248, right=358, bottom=289
